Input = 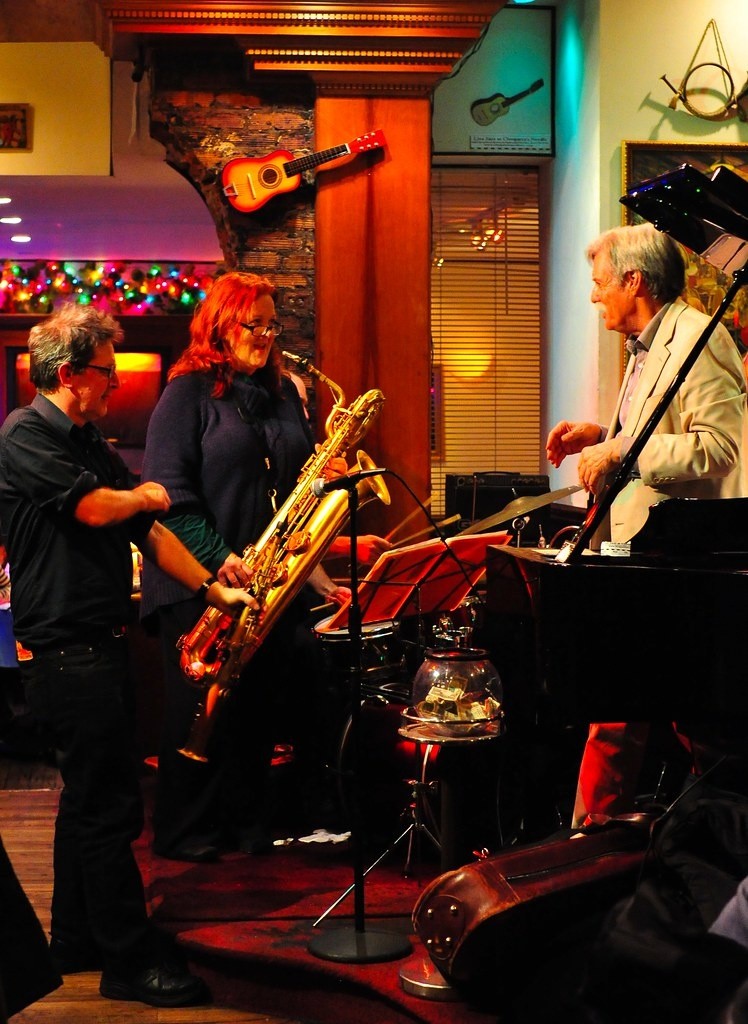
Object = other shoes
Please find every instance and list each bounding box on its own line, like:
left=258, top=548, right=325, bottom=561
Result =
left=165, top=844, right=217, bottom=862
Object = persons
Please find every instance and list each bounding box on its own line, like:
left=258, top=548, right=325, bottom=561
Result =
left=1, top=303, right=259, bottom=1009
left=546, top=226, right=748, bottom=831
left=140, top=271, right=355, bottom=862
left=282, top=531, right=394, bottom=607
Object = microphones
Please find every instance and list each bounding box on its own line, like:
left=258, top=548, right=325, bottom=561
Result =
left=310, top=468, right=387, bottom=499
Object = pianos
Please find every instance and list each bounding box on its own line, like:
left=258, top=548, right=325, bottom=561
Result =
left=474, top=158, right=747, bottom=817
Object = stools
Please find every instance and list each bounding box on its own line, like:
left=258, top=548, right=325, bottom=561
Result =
left=398, top=722, right=508, bottom=882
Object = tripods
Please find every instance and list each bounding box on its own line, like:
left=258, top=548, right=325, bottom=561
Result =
left=312, top=548, right=489, bottom=928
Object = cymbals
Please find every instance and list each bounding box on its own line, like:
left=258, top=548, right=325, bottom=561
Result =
left=454, top=482, right=585, bottom=535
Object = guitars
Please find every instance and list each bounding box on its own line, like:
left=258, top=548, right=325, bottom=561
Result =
left=221, top=128, right=387, bottom=215
left=471, top=77, right=545, bottom=127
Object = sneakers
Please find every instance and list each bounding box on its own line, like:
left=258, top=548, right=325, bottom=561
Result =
left=48, top=936, right=104, bottom=974
left=101, top=953, right=204, bottom=1006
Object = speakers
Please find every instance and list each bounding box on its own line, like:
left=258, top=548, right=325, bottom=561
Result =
left=445, top=473, right=550, bottom=546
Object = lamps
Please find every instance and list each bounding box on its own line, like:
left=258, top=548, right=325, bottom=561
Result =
left=470, top=212, right=506, bottom=252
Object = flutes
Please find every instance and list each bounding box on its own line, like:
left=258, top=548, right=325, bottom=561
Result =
left=176, top=513, right=310, bottom=766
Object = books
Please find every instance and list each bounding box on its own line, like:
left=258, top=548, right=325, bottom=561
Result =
left=327, top=530, right=513, bottom=629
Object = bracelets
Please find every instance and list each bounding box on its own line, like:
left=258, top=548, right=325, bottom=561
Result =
left=198, top=577, right=218, bottom=598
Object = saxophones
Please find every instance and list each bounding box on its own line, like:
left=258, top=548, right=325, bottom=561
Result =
left=175, top=348, right=386, bottom=679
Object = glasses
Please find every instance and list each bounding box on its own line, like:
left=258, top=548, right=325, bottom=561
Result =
left=83, top=363, right=116, bottom=378
left=240, top=319, right=284, bottom=337
